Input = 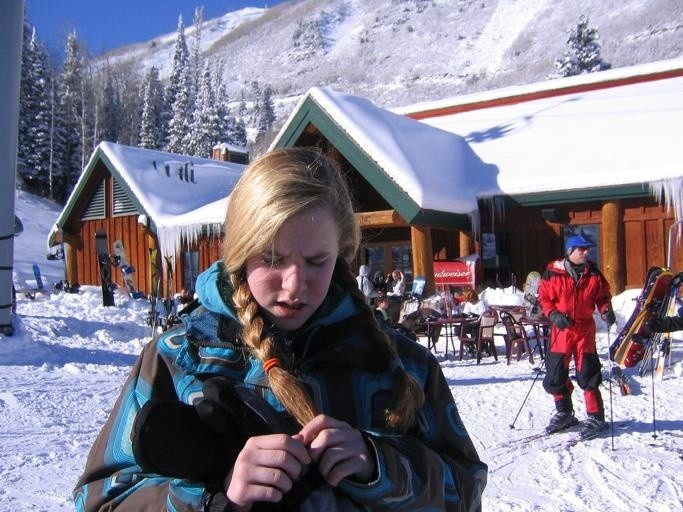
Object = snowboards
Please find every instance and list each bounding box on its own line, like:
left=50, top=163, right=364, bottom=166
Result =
left=112, top=239, right=142, bottom=302
left=14, top=286, right=49, bottom=295
left=94, top=229, right=116, bottom=306
left=400, top=276, right=426, bottom=342
left=521, top=271, right=541, bottom=316
left=33, top=265, right=43, bottom=291
left=55, top=224, right=71, bottom=292
left=609, top=267, right=673, bottom=367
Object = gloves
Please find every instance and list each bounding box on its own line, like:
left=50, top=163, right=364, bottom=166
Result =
left=550, top=309, right=574, bottom=331
left=600, top=305, right=616, bottom=325
left=645, top=316, right=661, bottom=333
left=129, top=374, right=328, bottom=511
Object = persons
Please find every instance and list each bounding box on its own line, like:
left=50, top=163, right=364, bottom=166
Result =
left=369, top=271, right=387, bottom=294
left=384, top=269, right=407, bottom=299
left=454, top=289, right=486, bottom=358
left=372, top=295, right=417, bottom=341
left=69, top=142, right=491, bottom=511
left=353, top=264, right=372, bottom=306
left=535, top=234, right=616, bottom=438
left=644, top=271, right=682, bottom=334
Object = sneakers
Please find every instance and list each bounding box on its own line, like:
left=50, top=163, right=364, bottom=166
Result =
left=578, top=414, right=610, bottom=441
left=544, top=411, right=578, bottom=435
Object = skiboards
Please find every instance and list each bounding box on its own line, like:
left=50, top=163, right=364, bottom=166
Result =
left=655, top=296, right=676, bottom=382
left=148, top=247, right=176, bottom=336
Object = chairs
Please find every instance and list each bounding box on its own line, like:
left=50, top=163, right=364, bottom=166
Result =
left=406, top=304, right=556, bottom=367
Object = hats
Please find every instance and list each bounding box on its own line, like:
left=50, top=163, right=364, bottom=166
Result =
left=565, top=235, right=592, bottom=255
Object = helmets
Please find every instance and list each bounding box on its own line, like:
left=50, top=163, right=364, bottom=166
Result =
left=670, top=272, right=683, bottom=286
